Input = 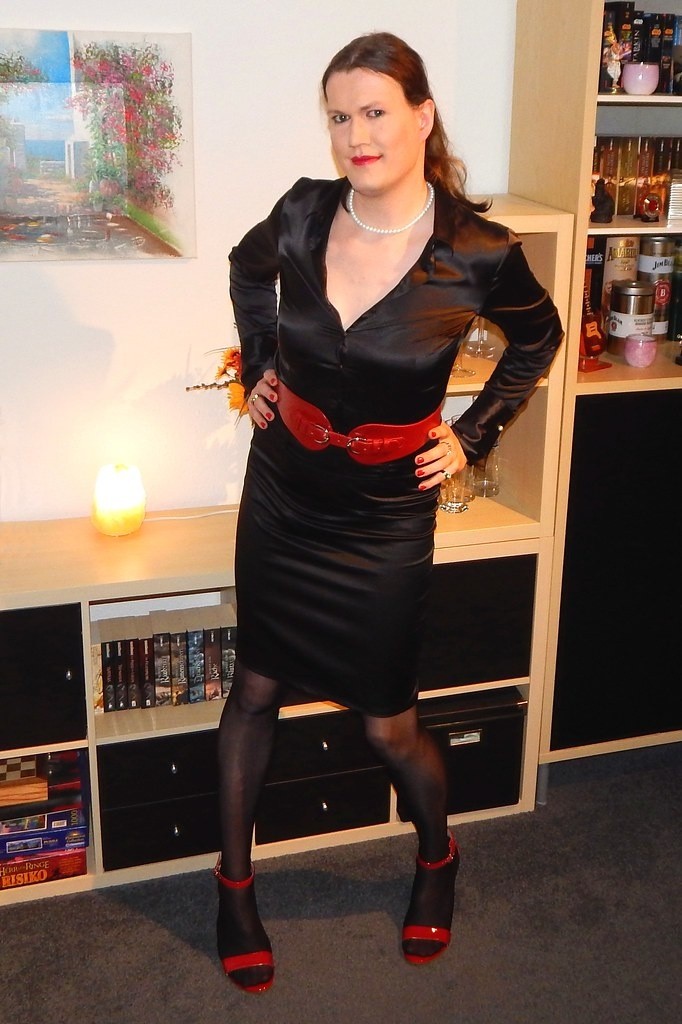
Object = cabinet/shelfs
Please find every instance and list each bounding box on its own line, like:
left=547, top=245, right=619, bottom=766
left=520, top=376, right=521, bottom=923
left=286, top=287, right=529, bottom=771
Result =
left=431, top=195, right=574, bottom=553
left=510, top=1, right=682, bottom=766
left=0, top=488, right=554, bottom=910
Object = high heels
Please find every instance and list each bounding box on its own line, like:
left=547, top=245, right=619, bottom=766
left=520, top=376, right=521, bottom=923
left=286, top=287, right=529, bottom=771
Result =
left=401, top=828, right=461, bottom=964
left=213, top=853, right=274, bottom=992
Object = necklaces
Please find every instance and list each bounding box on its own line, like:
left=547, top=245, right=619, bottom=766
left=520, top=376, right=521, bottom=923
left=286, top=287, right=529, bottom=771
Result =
left=348, top=180, right=433, bottom=233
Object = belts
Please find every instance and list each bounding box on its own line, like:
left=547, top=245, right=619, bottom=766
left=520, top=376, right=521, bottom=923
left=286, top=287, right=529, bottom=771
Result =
left=273, top=378, right=442, bottom=465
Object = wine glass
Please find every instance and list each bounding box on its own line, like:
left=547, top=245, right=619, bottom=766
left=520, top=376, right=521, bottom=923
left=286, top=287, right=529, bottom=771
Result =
left=451, top=312, right=497, bottom=378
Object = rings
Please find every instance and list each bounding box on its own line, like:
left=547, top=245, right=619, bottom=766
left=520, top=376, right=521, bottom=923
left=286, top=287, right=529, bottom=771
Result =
left=440, top=441, right=451, bottom=454
left=439, top=470, right=451, bottom=479
left=250, top=392, right=262, bottom=405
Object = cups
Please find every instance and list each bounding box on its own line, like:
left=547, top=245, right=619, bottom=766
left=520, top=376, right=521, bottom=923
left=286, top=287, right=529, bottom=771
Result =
left=621, top=60, right=659, bottom=96
left=438, top=394, right=502, bottom=514
left=593, top=132, right=681, bottom=216
left=607, top=278, right=655, bottom=355
left=639, top=237, right=681, bottom=341
left=625, top=335, right=655, bottom=370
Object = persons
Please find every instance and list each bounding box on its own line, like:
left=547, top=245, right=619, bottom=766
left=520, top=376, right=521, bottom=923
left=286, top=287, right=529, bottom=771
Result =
left=214, top=29, right=563, bottom=993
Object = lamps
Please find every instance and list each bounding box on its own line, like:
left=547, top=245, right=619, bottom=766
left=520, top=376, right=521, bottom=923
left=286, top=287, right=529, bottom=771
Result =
left=94, top=464, right=147, bottom=542
